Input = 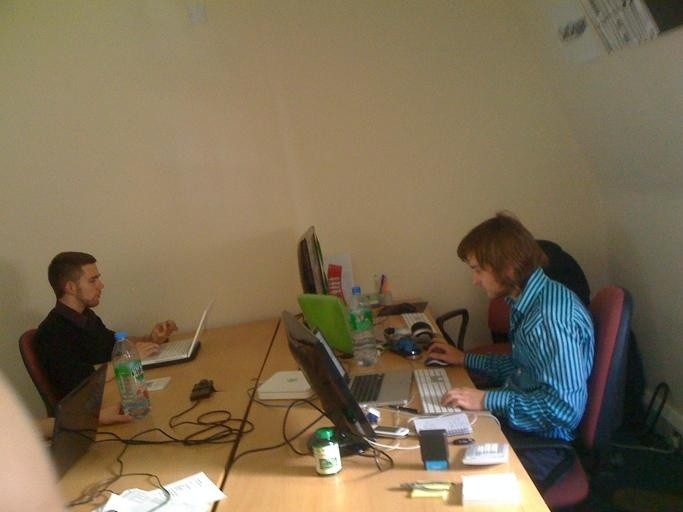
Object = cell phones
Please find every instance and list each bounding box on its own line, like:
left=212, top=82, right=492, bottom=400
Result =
left=371, top=425, right=409, bottom=438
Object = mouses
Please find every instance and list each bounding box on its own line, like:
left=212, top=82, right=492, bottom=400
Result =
left=424, top=357, right=449, bottom=367
left=392, top=303, right=416, bottom=314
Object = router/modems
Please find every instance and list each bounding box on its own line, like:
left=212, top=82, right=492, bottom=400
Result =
left=257, top=370, right=314, bottom=400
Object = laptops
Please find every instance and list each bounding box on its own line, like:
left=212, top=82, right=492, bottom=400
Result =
left=45, top=363, right=108, bottom=483
left=312, top=326, right=412, bottom=407
left=140, top=297, right=216, bottom=366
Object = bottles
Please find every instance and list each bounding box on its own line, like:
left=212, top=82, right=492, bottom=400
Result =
left=312, top=427, right=341, bottom=477
left=348, top=286, right=377, bottom=369
left=111, top=330, right=151, bottom=420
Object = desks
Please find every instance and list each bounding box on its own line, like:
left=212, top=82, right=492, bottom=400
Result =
left=211, top=297, right=554, bottom=510
left=40, top=296, right=285, bottom=511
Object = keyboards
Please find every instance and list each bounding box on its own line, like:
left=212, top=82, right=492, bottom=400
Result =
left=413, top=367, right=461, bottom=414
left=401, top=312, right=437, bottom=336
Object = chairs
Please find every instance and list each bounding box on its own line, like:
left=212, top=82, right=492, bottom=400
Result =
left=16, top=324, right=68, bottom=419
left=502, top=281, right=632, bottom=512
left=435, top=239, right=594, bottom=395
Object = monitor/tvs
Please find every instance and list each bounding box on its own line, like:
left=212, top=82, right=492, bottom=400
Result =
left=281, top=310, right=378, bottom=459
left=297, top=226, right=329, bottom=295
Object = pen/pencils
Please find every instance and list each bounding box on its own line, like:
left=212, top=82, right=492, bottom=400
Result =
left=374, top=274, right=387, bottom=293
left=389, top=404, right=419, bottom=414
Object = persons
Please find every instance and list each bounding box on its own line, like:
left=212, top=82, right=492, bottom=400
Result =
left=40, top=398, right=134, bottom=441
left=32, top=251, right=179, bottom=397
left=425, top=214, right=595, bottom=495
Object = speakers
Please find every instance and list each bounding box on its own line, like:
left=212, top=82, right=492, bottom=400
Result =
left=420, top=430, right=449, bottom=470
